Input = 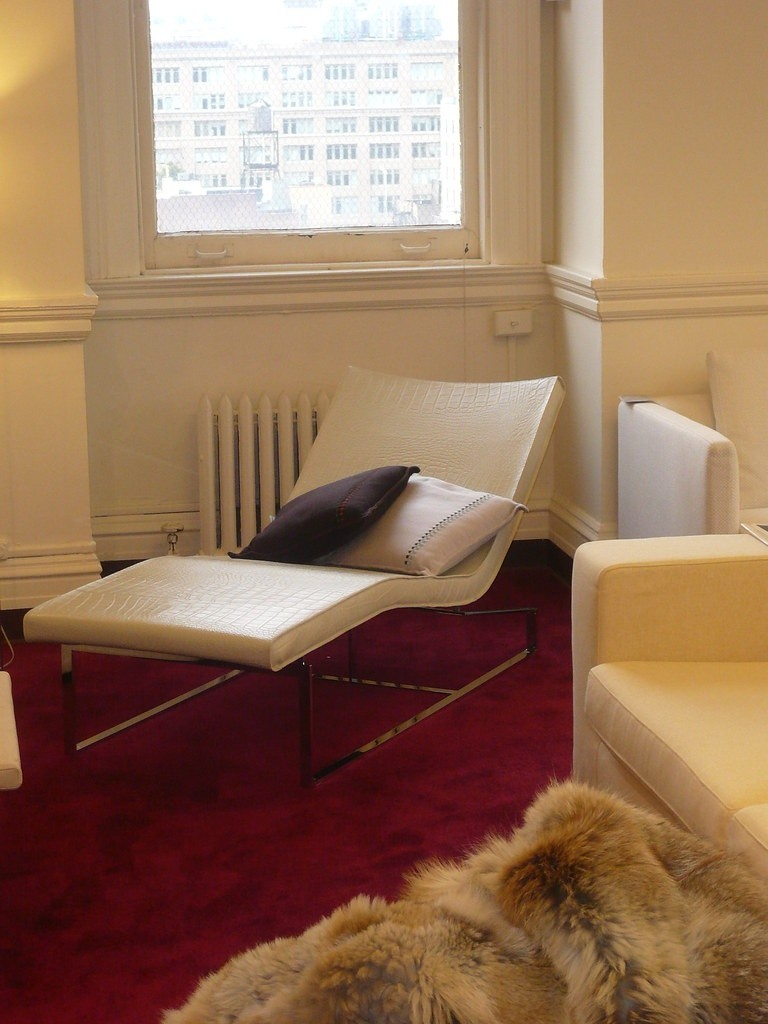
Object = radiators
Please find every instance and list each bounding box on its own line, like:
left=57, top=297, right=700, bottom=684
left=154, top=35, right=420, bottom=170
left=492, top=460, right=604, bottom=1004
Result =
left=196, top=388, right=332, bottom=556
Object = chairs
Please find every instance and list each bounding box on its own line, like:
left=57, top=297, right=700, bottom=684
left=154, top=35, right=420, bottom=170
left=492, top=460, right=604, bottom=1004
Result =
left=22, top=363, right=569, bottom=791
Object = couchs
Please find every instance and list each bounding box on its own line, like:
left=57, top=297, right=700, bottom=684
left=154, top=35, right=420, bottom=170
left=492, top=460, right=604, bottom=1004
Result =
left=571, top=534, right=768, bottom=878
left=616, top=394, right=768, bottom=538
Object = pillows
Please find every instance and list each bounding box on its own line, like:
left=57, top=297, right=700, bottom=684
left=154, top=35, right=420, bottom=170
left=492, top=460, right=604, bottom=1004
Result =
left=705, top=348, right=768, bottom=510
left=311, top=472, right=530, bottom=577
left=226, top=466, right=421, bottom=565
